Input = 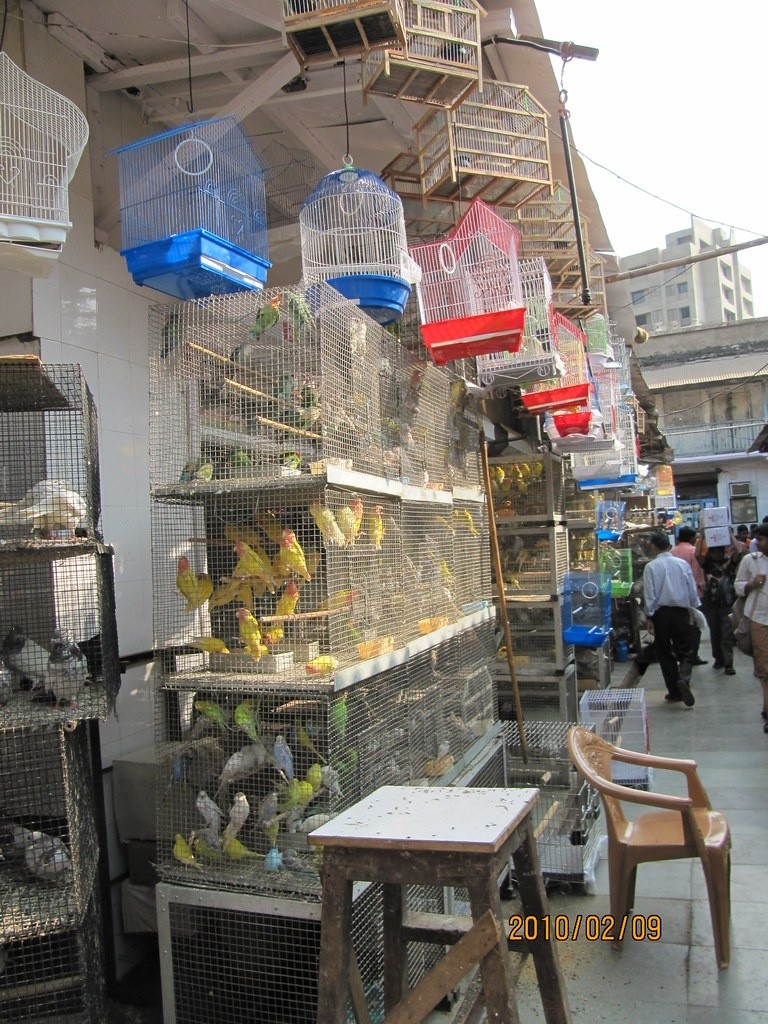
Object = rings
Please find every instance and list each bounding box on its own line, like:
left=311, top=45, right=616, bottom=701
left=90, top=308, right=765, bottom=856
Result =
left=760, top=582, right=762, bottom=584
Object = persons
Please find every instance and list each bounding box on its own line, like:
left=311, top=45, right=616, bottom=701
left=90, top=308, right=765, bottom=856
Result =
left=669, top=516, right=768, bottom=732
left=643, top=530, right=703, bottom=706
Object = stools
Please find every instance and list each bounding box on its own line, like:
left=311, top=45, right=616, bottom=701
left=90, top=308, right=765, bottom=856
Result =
left=306, top=785, right=571, bottom=1024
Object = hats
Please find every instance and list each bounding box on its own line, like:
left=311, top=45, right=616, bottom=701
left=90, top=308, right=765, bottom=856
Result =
left=679, top=526, right=696, bottom=540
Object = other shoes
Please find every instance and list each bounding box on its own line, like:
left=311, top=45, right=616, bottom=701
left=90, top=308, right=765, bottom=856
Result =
left=725, top=666, right=736, bottom=675
left=677, top=679, right=695, bottom=707
left=665, top=694, right=682, bottom=702
left=713, top=660, right=722, bottom=668
left=694, top=656, right=708, bottom=665
left=762, top=711, right=768, bottom=733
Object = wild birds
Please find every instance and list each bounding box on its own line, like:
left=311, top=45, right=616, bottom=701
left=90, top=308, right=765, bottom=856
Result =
left=1, top=821, right=71, bottom=893
left=1, top=625, right=89, bottom=716
left=158, top=288, right=655, bottom=882
left=1, top=477, right=86, bottom=542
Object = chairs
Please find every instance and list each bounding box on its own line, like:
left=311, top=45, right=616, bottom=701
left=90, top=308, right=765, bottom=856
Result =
left=568, top=725, right=734, bottom=969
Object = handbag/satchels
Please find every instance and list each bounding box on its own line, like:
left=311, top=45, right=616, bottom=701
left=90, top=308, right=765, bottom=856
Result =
left=728, top=595, right=754, bottom=656
